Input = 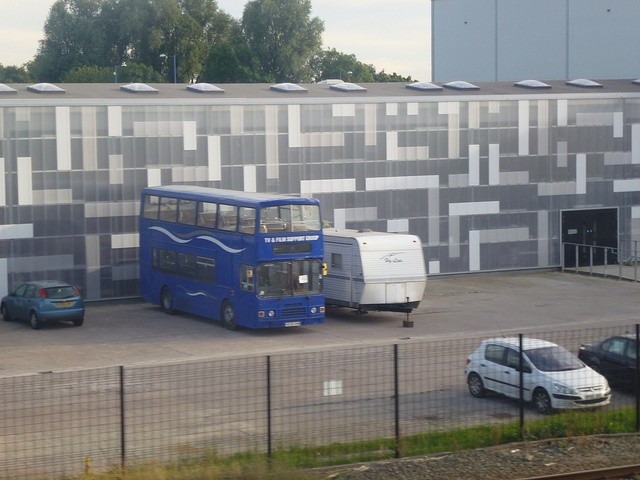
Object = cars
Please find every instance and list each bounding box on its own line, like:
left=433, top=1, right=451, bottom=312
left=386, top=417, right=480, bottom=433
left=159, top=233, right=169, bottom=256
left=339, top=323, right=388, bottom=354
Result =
left=1, top=280, right=84, bottom=328
left=579, top=336, right=638, bottom=390
left=464, top=337, right=612, bottom=413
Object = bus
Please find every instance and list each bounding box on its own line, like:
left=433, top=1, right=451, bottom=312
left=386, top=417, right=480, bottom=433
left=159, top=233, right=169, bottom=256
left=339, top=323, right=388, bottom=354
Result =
left=139, top=185, right=328, bottom=330
